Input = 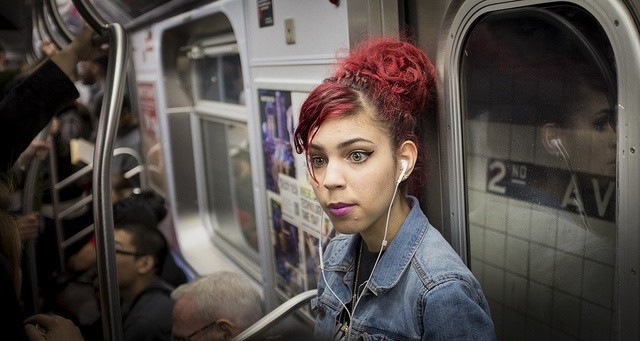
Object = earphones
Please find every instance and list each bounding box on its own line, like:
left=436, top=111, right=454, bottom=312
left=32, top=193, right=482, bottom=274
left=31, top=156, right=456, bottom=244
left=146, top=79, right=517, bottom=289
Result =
left=401, top=161, right=408, bottom=171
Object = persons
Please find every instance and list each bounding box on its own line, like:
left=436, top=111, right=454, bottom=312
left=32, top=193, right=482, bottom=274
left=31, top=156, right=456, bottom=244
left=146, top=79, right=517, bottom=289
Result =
left=111, top=174, right=168, bottom=228
left=172, top=273, right=268, bottom=341
left=294, top=38, right=495, bottom=340
left=0, top=173, right=85, bottom=341
left=1, top=24, right=138, bottom=204
left=94, top=223, right=173, bottom=340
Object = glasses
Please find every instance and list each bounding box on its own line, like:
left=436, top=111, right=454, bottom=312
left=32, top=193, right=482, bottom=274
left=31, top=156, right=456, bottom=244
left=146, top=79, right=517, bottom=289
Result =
left=113, top=242, right=140, bottom=261
left=175, top=322, right=216, bottom=341
